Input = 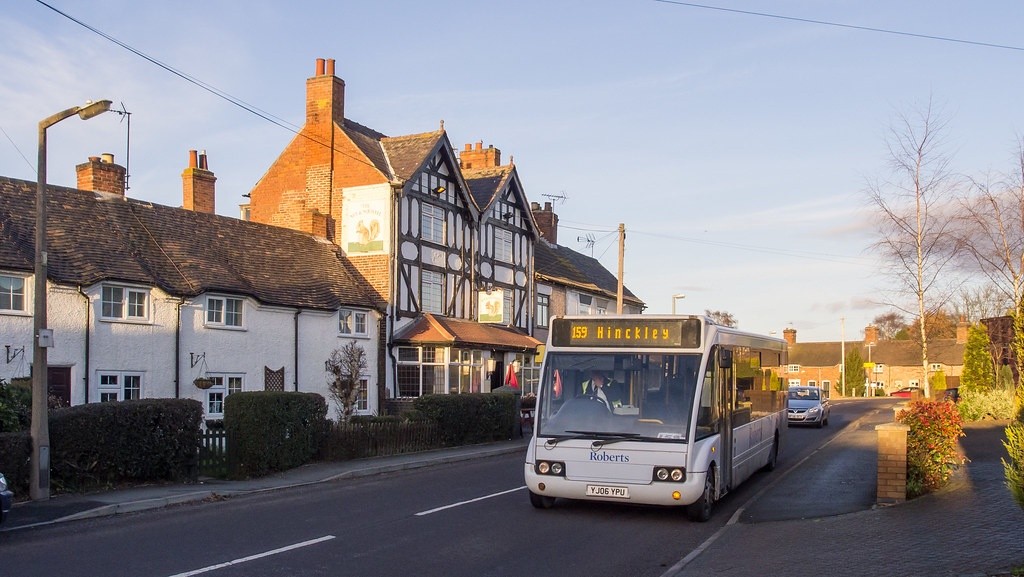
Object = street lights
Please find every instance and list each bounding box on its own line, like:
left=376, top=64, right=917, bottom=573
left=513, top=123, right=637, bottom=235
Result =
left=671, top=293, right=686, bottom=315
left=29, top=99, right=114, bottom=501
left=768, top=329, right=777, bottom=336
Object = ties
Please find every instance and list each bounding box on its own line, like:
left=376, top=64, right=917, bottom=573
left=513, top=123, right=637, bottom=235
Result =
left=592, top=385, right=598, bottom=401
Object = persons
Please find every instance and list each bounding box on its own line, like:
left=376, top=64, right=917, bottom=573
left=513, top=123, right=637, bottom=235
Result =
left=576, top=370, right=623, bottom=412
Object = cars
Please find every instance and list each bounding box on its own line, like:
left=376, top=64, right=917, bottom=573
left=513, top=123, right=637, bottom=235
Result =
left=890, top=386, right=920, bottom=397
left=786, top=386, right=831, bottom=429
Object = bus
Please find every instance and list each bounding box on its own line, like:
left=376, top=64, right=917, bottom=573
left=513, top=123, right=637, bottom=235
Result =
left=523, top=313, right=790, bottom=524
